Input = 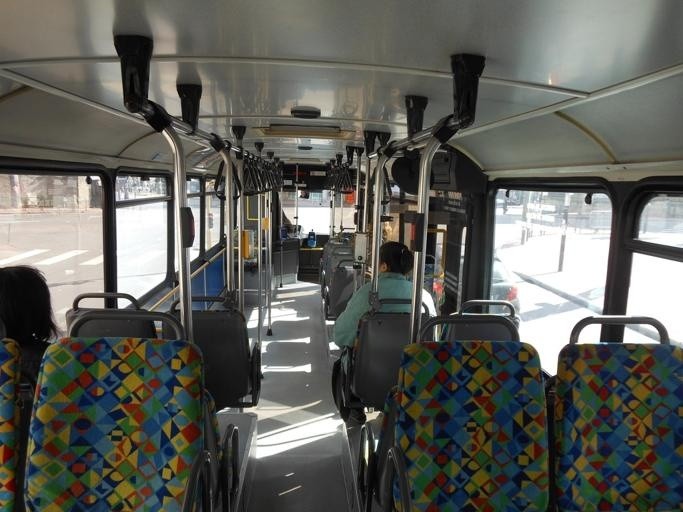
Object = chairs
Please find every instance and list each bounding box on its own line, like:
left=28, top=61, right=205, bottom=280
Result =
left=318, top=234, right=682, bottom=512
left=1, top=293, right=262, bottom=511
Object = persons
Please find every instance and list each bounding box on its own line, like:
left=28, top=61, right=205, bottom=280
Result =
left=329, top=241, right=438, bottom=427
left=299, top=191, right=308, bottom=198
